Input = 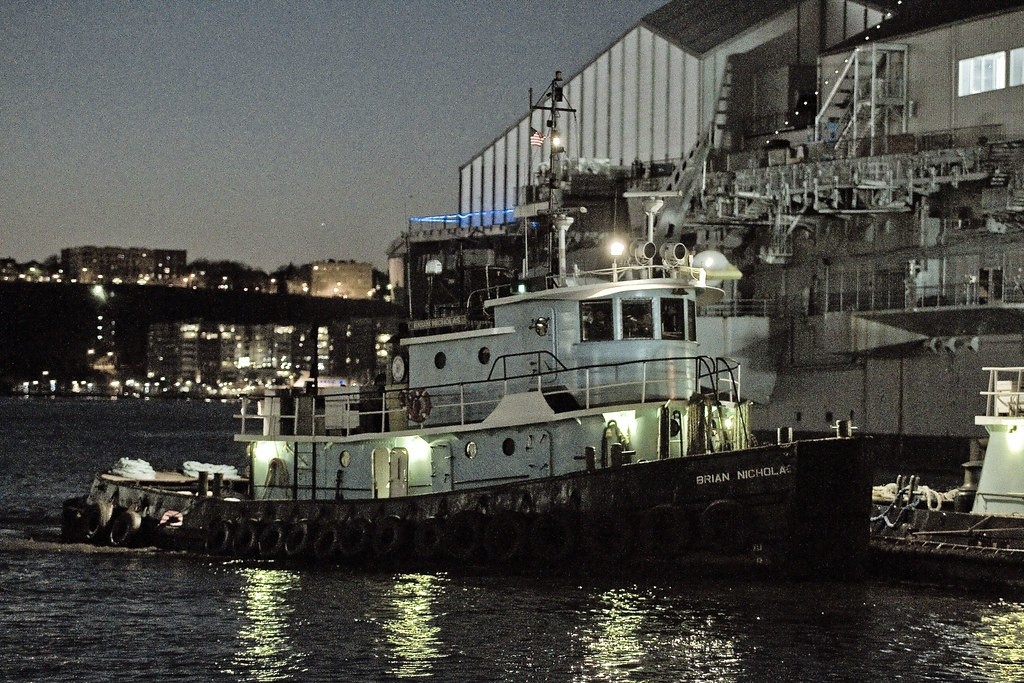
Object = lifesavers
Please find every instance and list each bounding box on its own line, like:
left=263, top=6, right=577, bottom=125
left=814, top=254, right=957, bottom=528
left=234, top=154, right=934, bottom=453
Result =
left=81, top=501, right=113, bottom=543
left=373, top=514, right=405, bottom=558
left=637, top=504, right=692, bottom=559
left=579, top=509, right=636, bottom=561
left=107, top=511, right=142, bottom=548
left=407, top=389, right=432, bottom=423
left=698, top=499, right=754, bottom=556
left=233, top=517, right=258, bottom=557
left=409, top=518, right=449, bottom=560
left=339, top=518, right=373, bottom=559
left=205, top=518, right=232, bottom=554
left=446, top=509, right=487, bottom=559
left=313, top=522, right=342, bottom=560
left=484, top=510, right=530, bottom=561
left=257, top=517, right=287, bottom=558
left=285, top=518, right=315, bottom=560
left=530, top=513, right=577, bottom=561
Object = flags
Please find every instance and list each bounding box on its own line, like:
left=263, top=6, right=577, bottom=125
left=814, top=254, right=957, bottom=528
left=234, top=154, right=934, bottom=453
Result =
left=529, top=125, right=545, bottom=146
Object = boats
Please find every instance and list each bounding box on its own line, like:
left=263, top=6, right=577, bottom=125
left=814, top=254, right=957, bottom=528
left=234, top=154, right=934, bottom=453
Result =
left=867, top=368, right=1024, bottom=599
left=58, top=70, right=880, bottom=583
